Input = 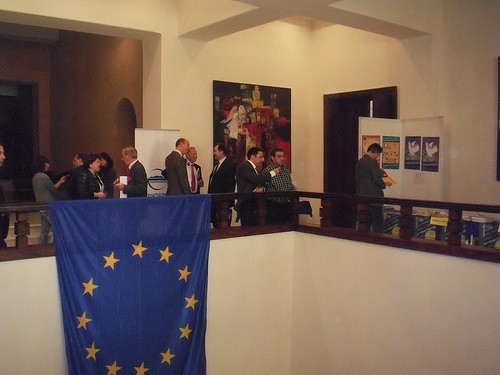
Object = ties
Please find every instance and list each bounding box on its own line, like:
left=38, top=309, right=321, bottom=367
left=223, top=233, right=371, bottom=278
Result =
left=213, top=161, right=219, bottom=174
left=191, top=164, right=196, bottom=192
left=126, top=167, right=130, bottom=173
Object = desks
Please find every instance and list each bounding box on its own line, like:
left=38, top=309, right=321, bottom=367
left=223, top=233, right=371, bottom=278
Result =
left=383, top=206, right=500, bottom=248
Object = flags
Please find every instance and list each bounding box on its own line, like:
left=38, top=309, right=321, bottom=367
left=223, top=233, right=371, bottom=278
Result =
left=49, top=193, right=211, bottom=375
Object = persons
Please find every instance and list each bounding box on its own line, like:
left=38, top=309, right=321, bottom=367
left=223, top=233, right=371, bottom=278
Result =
left=31, top=137, right=296, bottom=243
left=0, top=144, right=10, bottom=248
left=354, top=143, right=386, bottom=232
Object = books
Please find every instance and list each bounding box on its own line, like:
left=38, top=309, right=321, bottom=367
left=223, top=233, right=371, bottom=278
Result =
left=472, top=215, right=494, bottom=223
